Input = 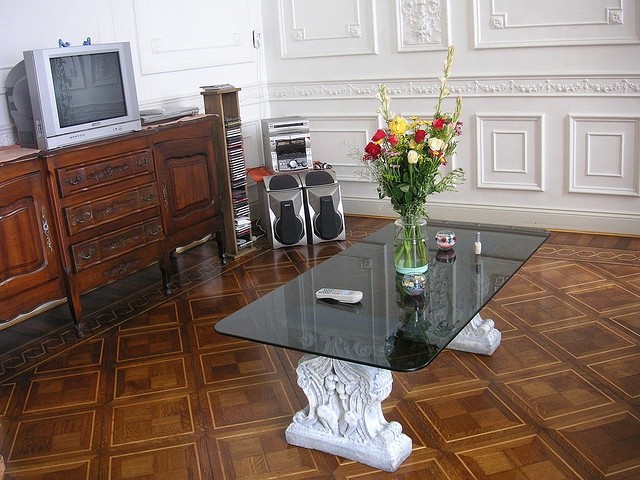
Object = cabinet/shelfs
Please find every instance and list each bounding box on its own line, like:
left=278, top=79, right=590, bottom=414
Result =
left=200, top=86, right=257, bottom=259
left=40, top=128, right=174, bottom=337
left=151, top=114, right=227, bottom=295
left=1, top=145, right=68, bottom=331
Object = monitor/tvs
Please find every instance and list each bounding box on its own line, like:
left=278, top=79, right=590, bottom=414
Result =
left=5, top=42, right=143, bottom=151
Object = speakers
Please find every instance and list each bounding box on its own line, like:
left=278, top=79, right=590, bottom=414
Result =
left=296, top=169, right=346, bottom=245
left=257, top=173, right=308, bottom=250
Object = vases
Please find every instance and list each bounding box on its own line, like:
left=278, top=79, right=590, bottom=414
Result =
left=394, top=219, right=428, bottom=274
left=341, top=47, right=466, bottom=270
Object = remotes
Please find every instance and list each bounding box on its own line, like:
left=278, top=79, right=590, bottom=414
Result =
left=315, top=287, right=363, bottom=304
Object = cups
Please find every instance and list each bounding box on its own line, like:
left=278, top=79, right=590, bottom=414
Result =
left=401, top=272, right=427, bottom=296
left=434, top=230, right=456, bottom=251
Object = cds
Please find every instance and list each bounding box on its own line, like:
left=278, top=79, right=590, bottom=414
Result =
left=236, top=238, right=247, bottom=245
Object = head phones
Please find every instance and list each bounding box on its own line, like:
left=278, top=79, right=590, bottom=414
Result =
left=314, top=161, right=332, bottom=170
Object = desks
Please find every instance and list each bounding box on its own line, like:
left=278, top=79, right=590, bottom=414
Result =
left=213, top=217, right=550, bottom=473
